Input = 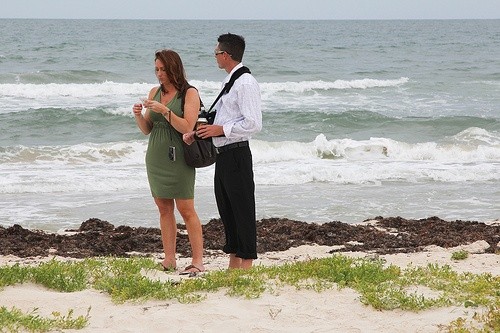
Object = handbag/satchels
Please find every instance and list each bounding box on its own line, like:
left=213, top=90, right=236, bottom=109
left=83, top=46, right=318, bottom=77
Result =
left=183, top=86, right=216, bottom=167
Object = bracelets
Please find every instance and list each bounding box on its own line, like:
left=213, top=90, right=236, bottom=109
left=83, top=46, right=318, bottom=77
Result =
left=163, top=110, right=170, bottom=116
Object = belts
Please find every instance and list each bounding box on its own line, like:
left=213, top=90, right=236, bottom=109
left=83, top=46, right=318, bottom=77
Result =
left=217, top=141, right=248, bottom=154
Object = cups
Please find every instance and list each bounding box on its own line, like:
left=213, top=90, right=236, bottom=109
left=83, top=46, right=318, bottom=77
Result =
left=197, top=118, right=208, bottom=135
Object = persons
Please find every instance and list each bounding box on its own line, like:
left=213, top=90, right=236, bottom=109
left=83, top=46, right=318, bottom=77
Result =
left=182, top=32, right=265, bottom=271
left=132, top=49, right=207, bottom=275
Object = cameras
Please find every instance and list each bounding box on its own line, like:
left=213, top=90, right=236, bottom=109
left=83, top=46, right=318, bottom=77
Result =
left=168, top=146, right=175, bottom=161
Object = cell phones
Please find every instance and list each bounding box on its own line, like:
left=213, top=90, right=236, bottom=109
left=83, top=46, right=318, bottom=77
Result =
left=140, top=97, right=148, bottom=104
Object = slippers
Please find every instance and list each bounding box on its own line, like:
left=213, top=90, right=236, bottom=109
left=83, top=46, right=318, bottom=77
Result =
left=184, top=265, right=209, bottom=274
left=159, top=264, right=178, bottom=271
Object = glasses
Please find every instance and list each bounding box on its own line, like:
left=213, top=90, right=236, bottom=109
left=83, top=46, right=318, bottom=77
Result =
left=214, top=49, right=232, bottom=58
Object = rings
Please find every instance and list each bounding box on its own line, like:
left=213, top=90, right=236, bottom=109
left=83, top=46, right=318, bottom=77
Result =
left=148, top=107, right=151, bottom=109
